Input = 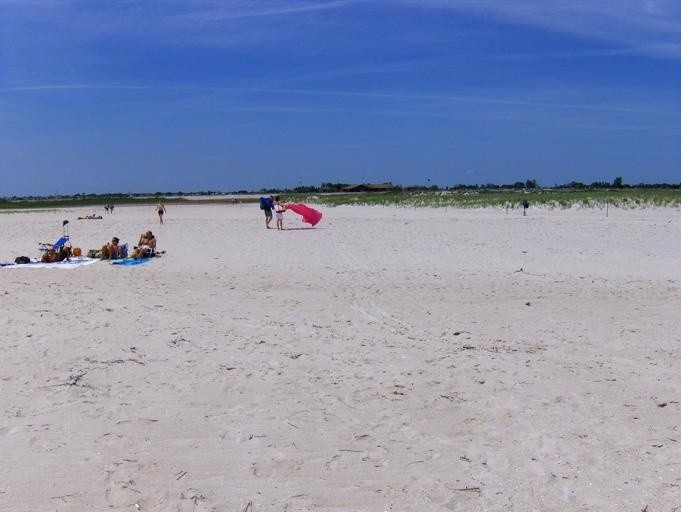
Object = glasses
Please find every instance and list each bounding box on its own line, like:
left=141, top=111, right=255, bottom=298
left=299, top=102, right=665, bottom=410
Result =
left=112, top=239, right=117, bottom=243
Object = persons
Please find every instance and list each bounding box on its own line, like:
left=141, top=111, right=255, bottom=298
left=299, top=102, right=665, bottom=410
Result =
left=105, top=202, right=114, bottom=214
left=77, top=214, right=103, bottom=219
left=133, top=231, right=156, bottom=260
left=155, top=199, right=166, bottom=224
left=100, top=237, right=120, bottom=261
left=41, top=246, right=73, bottom=263
left=264, top=196, right=285, bottom=230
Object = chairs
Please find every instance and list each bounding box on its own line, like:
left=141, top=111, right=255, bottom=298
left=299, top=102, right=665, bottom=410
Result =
left=37, top=234, right=69, bottom=259
left=138, top=234, right=157, bottom=259
